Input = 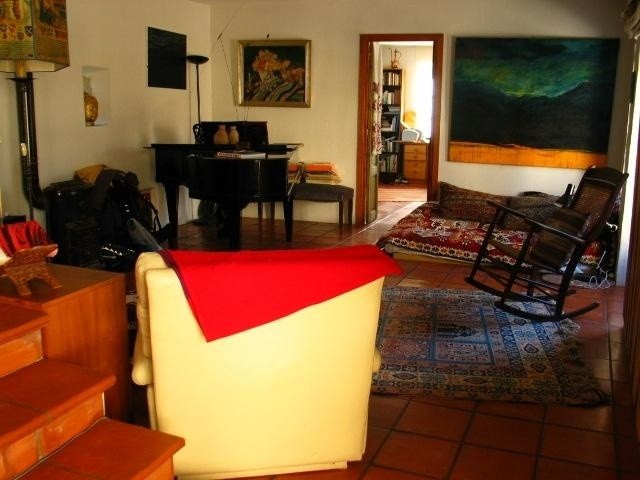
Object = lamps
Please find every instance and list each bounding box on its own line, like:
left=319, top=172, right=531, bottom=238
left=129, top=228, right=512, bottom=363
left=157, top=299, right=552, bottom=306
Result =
left=0, top=0, right=71, bottom=219
left=185, top=53, right=210, bottom=225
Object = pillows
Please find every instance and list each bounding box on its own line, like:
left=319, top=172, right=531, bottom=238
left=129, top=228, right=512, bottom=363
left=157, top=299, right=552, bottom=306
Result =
left=502, top=195, right=564, bottom=230
left=438, top=181, right=507, bottom=222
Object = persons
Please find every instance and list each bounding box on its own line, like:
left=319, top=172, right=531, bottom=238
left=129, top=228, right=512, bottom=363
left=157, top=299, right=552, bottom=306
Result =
left=84, top=92, right=99, bottom=127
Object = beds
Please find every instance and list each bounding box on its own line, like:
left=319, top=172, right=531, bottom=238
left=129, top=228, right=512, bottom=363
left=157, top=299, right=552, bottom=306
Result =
left=376, top=199, right=618, bottom=281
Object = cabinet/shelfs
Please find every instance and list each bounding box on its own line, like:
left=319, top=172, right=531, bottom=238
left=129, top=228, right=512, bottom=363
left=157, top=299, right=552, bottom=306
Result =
left=377, top=69, right=405, bottom=185
left=403, top=144, right=429, bottom=184
left=0, top=259, right=131, bottom=426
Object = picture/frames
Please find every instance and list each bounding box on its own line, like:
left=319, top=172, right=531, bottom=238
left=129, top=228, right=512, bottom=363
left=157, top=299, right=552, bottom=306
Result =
left=234, top=38, right=312, bottom=107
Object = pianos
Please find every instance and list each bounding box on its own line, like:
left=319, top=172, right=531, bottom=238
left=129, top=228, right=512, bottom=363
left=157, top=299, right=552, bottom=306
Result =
left=151, top=121, right=303, bottom=248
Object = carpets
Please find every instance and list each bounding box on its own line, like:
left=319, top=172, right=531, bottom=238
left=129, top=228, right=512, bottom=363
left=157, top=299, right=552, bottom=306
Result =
left=368, top=287, right=607, bottom=408
left=377, top=187, right=427, bottom=203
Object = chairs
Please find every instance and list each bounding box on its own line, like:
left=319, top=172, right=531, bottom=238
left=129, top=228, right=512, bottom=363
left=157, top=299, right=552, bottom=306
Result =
left=465, top=166, right=632, bottom=325
left=127, top=244, right=388, bottom=479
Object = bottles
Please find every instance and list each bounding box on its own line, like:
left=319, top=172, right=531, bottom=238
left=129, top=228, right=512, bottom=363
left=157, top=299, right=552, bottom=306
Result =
left=228, top=126, right=240, bottom=144
left=213, top=125, right=229, bottom=144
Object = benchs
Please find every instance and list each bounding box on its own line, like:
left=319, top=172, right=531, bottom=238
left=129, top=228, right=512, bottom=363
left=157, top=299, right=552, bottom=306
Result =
left=255, top=183, right=354, bottom=228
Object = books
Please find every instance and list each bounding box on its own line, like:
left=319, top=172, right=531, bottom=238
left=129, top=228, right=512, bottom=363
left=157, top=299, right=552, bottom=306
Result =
left=287, top=161, right=340, bottom=186
left=216, top=149, right=265, bottom=159
left=379, top=71, right=400, bottom=173
